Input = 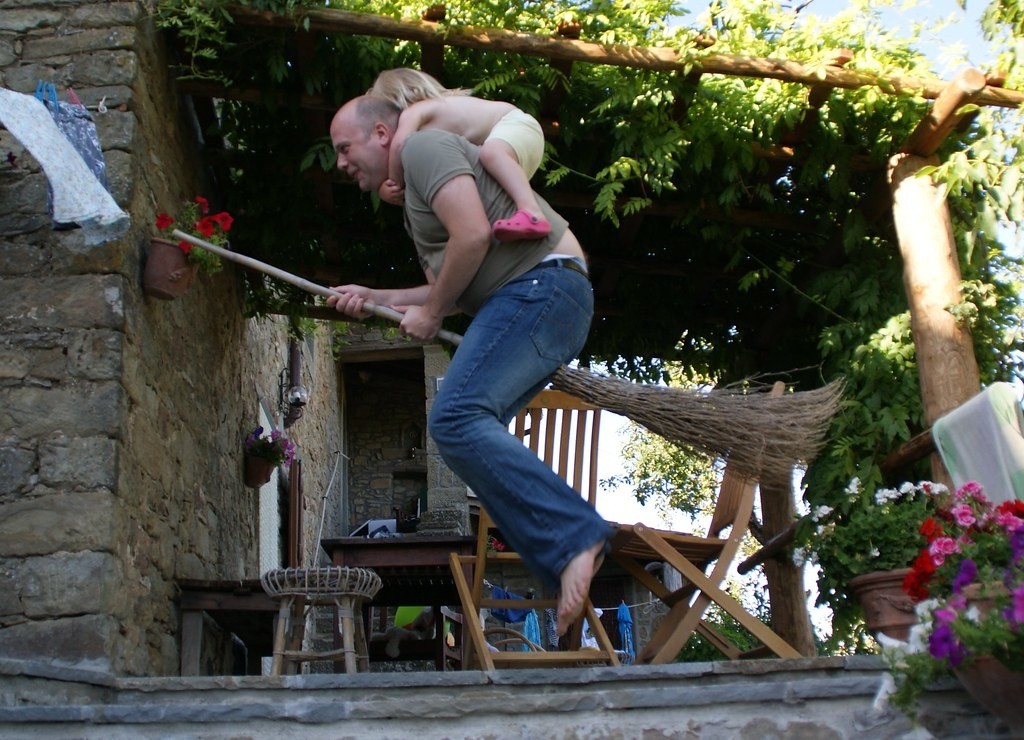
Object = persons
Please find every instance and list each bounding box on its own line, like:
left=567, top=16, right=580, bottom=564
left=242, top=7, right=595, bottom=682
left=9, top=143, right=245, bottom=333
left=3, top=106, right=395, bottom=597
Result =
left=326, top=96, right=614, bottom=635
left=370, top=67, right=553, bottom=241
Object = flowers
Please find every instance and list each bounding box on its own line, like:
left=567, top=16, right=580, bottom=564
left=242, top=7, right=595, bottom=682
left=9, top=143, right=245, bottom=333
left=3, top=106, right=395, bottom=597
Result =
left=156, top=194, right=234, bottom=276
left=245, top=425, right=297, bottom=468
left=790, top=477, right=1023, bottom=717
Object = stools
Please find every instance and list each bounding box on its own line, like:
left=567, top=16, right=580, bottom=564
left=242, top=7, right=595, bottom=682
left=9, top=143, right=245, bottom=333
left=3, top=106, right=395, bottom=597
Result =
left=260, top=564, right=384, bottom=675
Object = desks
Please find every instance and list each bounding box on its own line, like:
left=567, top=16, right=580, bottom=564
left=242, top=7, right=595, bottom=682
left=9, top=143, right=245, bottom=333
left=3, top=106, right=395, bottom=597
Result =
left=319, top=536, right=479, bottom=671
left=179, top=577, right=280, bottom=675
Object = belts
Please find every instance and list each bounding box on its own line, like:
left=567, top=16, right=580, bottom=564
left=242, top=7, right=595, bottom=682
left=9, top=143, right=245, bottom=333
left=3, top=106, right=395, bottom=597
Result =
left=538, top=259, right=590, bottom=279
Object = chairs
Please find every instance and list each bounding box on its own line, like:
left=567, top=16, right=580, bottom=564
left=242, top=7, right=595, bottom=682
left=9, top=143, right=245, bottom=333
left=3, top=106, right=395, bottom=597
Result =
left=447, top=389, right=622, bottom=671
left=604, top=380, right=804, bottom=665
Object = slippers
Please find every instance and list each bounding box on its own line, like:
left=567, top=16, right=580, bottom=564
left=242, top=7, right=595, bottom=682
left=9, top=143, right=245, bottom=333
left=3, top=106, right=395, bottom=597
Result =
left=493, top=210, right=551, bottom=239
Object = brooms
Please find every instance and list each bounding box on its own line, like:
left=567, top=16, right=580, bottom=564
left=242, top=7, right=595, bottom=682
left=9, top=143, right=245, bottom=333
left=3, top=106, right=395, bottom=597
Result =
left=171, top=227, right=849, bottom=493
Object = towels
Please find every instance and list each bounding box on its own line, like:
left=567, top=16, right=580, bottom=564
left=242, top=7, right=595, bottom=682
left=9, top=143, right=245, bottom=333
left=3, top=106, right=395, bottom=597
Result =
left=932, top=380, right=1024, bottom=507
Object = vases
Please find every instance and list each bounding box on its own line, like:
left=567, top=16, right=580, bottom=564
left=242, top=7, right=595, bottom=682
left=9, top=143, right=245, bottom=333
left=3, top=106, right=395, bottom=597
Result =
left=960, top=581, right=1012, bottom=621
left=849, top=568, right=919, bottom=642
left=142, top=238, right=203, bottom=301
left=243, top=456, right=275, bottom=489
left=948, top=655, right=1023, bottom=737
